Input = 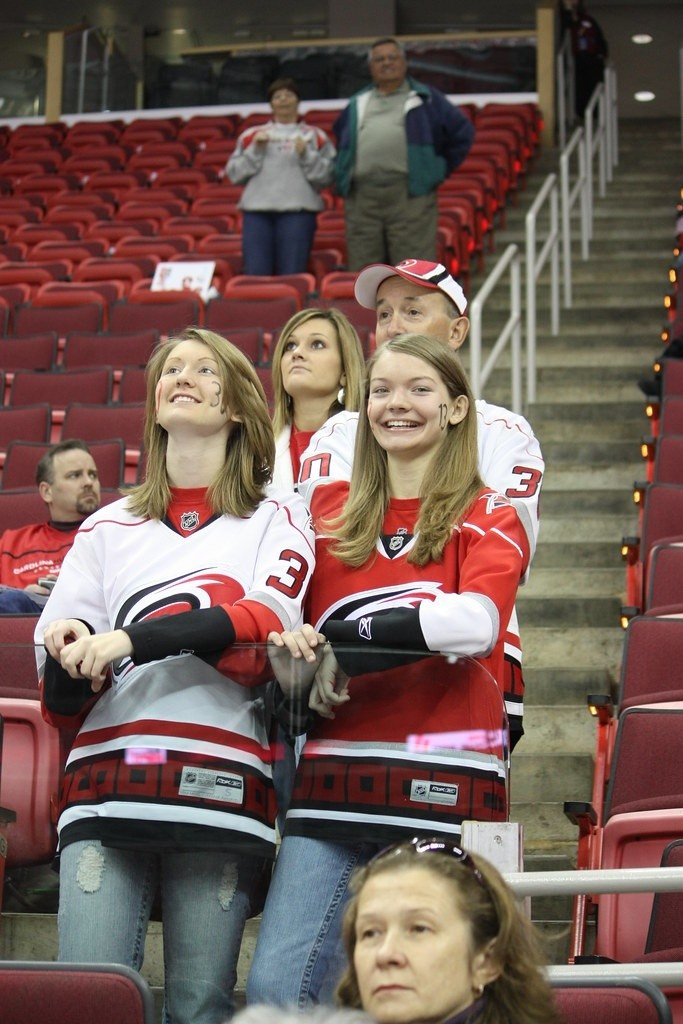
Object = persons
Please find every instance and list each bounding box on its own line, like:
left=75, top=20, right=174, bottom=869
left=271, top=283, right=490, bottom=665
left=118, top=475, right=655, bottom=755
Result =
left=34, top=330, right=314, bottom=1024
left=271, top=307, right=365, bottom=489
left=638, top=335, right=683, bottom=395
left=672, top=211, right=683, bottom=270
left=333, top=39, right=475, bottom=270
left=226, top=81, right=337, bottom=277
left=337, top=842, right=562, bottom=1024
left=559, top=0, right=609, bottom=130
left=354, top=258, right=544, bottom=764
left=182, top=276, right=193, bottom=292
left=0, top=439, right=101, bottom=616
left=245, top=333, right=534, bottom=1006
left=409, top=12, right=512, bottom=95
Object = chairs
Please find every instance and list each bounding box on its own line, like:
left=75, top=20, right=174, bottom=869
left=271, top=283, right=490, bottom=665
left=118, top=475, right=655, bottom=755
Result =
left=0, top=43, right=683, bottom=1021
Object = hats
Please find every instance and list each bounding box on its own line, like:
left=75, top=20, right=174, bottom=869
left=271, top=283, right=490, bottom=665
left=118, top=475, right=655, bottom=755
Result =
left=354, top=258, right=468, bottom=320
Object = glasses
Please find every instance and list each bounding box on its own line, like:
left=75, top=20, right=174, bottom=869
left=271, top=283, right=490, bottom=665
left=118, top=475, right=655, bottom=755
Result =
left=367, top=836, right=487, bottom=891
